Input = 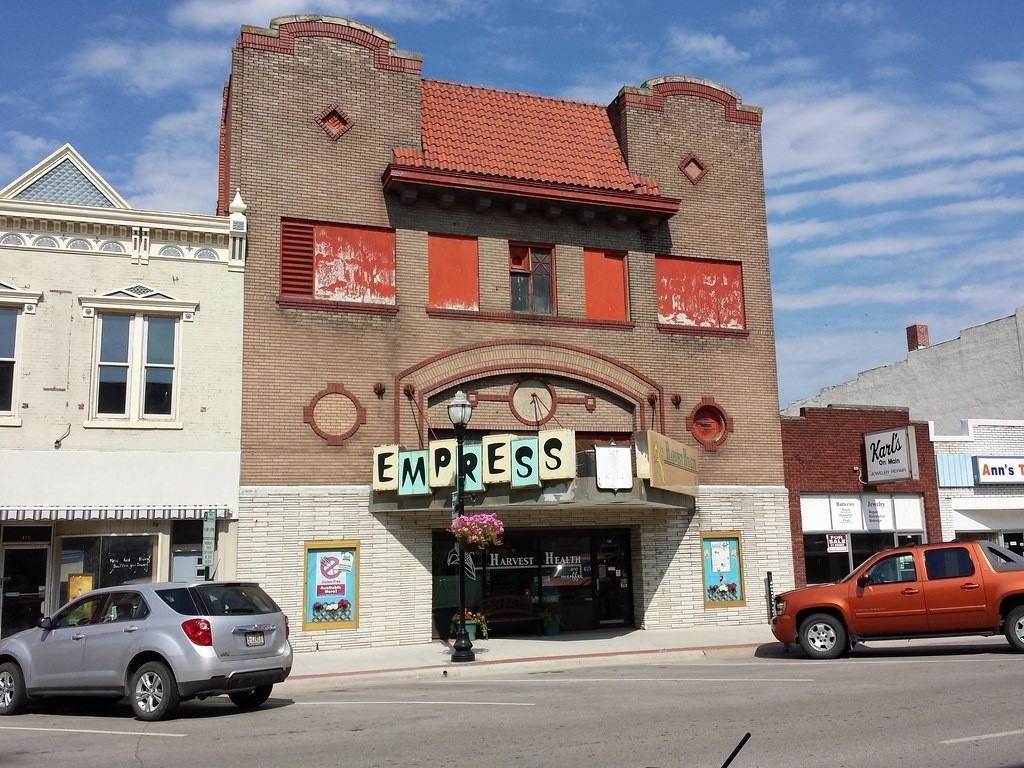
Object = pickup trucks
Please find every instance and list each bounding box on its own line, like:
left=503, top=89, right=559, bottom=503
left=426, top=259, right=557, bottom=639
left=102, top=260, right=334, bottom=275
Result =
left=770, top=542, right=1024, bottom=659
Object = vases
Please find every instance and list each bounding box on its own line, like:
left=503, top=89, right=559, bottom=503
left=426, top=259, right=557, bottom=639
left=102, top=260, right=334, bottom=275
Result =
left=457, top=619, right=476, bottom=640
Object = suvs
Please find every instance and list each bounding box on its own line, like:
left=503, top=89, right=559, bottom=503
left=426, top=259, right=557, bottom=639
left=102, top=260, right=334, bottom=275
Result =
left=1, top=582, right=291, bottom=718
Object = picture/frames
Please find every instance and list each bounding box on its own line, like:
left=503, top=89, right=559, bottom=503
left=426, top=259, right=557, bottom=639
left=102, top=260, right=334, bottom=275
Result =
left=66, top=573, right=97, bottom=620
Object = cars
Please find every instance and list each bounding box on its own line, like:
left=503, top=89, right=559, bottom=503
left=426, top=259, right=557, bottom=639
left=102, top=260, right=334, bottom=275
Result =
left=541, top=556, right=605, bottom=597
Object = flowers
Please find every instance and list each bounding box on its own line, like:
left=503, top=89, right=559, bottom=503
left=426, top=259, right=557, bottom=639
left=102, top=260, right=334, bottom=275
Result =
left=445, top=512, right=505, bottom=549
left=449, top=607, right=489, bottom=640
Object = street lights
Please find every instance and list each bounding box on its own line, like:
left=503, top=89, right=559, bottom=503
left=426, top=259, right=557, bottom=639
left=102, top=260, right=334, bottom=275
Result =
left=448, top=391, right=473, bottom=663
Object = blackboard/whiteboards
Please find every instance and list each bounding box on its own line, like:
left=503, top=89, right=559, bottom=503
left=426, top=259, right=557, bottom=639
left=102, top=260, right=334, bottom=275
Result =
left=102, top=552, right=153, bottom=586
